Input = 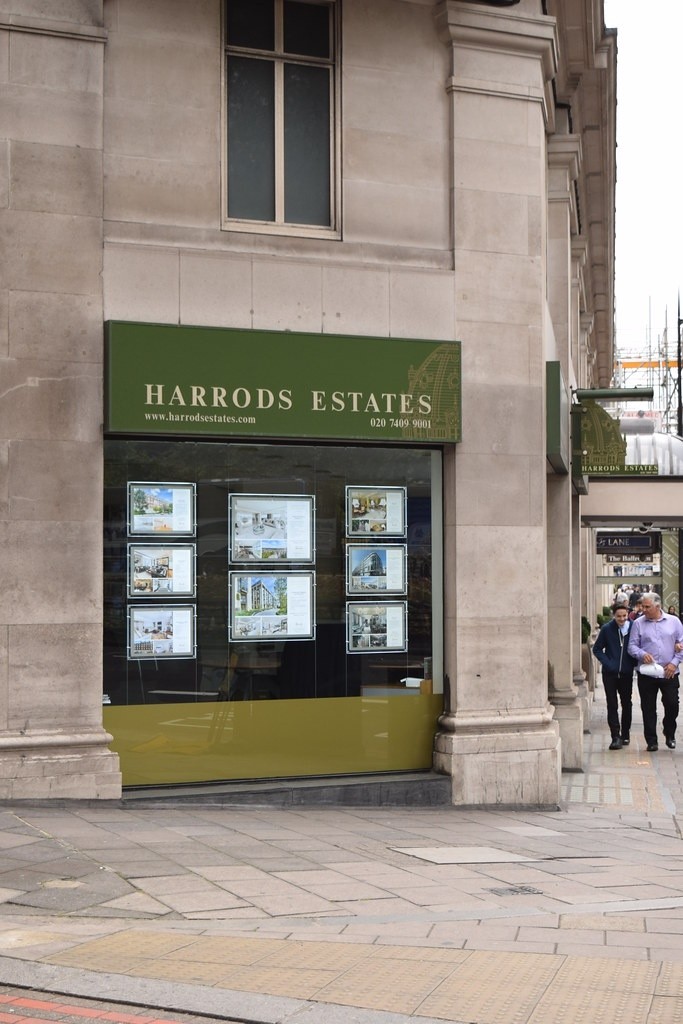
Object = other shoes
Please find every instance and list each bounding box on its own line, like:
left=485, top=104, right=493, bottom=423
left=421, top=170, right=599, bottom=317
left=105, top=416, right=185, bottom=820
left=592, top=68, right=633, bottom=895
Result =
left=621, top=729, right=630, bottom=744
left=609, top=735, right=623, bottom=749
left=663, top=729, right=676, bottom=748
left=647, top=742, right=658, bottom=751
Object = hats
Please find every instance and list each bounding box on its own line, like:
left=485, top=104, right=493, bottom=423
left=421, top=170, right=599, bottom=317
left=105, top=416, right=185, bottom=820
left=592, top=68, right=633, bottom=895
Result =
left=617, top=593, right=627, bottom=602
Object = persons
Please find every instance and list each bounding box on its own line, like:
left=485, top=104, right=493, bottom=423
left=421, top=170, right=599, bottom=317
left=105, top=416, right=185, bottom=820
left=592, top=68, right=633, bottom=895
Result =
left=592, top=584, right=683, bottom=752
